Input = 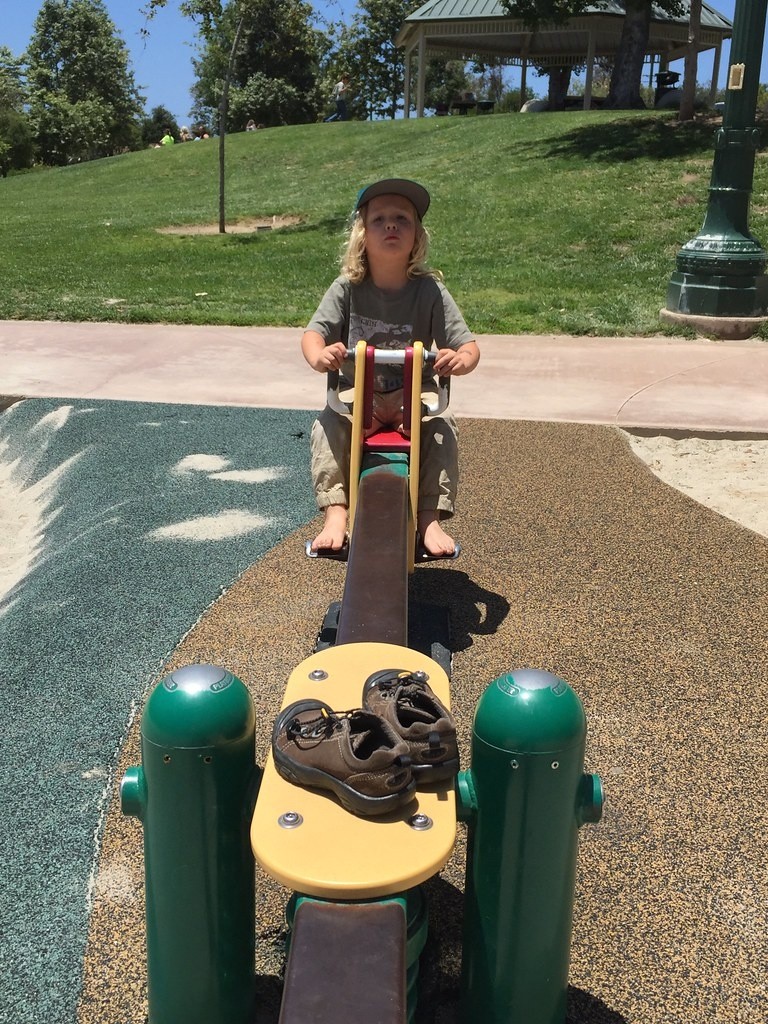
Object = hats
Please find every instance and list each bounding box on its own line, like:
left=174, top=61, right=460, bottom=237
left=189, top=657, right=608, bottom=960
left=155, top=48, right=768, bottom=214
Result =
left=352, top=178, right=431, bottom=224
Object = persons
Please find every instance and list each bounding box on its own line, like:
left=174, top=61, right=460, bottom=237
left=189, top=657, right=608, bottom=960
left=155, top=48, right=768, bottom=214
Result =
left=246, top=119, right=256, bottom=131
left=151, top=140, right=162, bottom=147
left=325, top=72, right=350, bottom=123
left=112, top=145, right=132, bottom=156
left=160, top=129, right=174, bottom=146
left=179, top=126, right=190, bottom=142
left=301, top=179, right=481, bottom=557
left=198, top=126, right=208, bottom=140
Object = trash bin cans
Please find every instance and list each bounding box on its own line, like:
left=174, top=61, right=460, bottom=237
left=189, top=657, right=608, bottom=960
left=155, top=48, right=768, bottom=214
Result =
left=476, top=99, right=496, bottom=115
left=436, top=103, right=450, bottom=116
left=654, top=69, right=681, bottom=109
